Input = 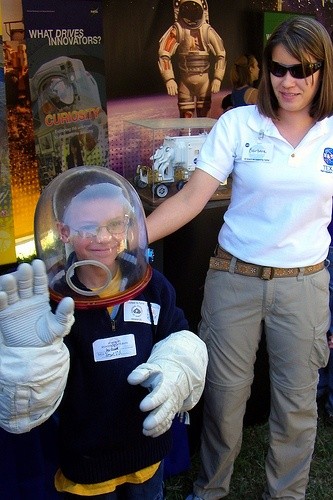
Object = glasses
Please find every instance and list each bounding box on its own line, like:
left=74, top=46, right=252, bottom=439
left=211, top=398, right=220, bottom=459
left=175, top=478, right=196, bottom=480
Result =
left=268, top=59, right=322, bottom=79
left=67, top=215, right=130, bottom=238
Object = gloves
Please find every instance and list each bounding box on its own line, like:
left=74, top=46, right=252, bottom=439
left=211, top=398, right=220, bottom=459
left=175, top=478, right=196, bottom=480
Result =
left=128, top=330, right=207, bottom=438
left=0, top=259, right=75, bottom=434
left=210, top=78, right=221, bottom=94
left=165, top=79, right=178, bottom=96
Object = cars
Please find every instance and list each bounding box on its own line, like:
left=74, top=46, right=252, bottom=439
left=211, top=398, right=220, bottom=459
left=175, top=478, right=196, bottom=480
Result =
left=132, top=131, right=227, bottom=199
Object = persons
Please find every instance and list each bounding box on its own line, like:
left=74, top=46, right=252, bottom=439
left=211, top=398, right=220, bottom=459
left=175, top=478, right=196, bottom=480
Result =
left=158, top=0, right=227, bottom=136
left=131, top=17, right=333, bottom=500
left=230, top=54, right=261, bottom=108
left=0, top=167, right=210, bottom=500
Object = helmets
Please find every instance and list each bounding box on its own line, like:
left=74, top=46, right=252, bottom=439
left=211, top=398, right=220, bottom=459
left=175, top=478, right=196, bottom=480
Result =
left=172, top=0, right=210, bottom=30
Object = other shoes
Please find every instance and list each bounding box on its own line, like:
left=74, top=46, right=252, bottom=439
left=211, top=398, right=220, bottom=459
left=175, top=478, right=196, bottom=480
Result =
left=184, top=494, right=205, bottom=500
